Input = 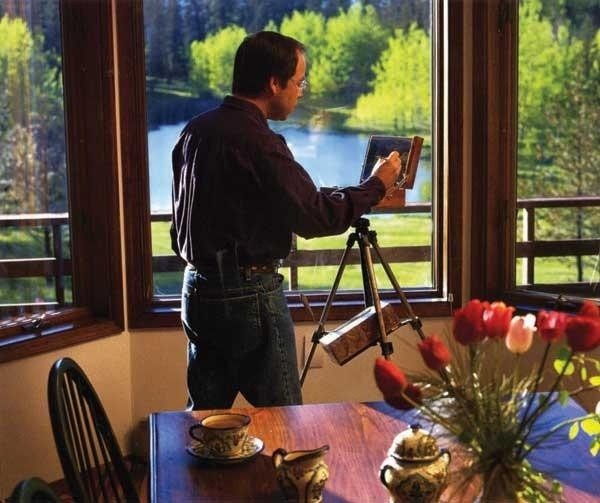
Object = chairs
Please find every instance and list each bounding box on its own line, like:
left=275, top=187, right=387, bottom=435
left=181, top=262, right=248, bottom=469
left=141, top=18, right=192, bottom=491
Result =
left=9, top=477, right=62, bottom=503
left=48, top=358, right=140, bottom=503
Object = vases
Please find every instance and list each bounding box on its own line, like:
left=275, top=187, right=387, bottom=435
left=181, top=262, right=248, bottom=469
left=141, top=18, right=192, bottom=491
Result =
left=484, top=472, right=516, bottom=502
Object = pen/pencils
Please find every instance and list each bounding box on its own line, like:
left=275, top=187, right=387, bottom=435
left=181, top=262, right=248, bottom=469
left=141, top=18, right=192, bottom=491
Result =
left=377, top=156, right=387, bottom=160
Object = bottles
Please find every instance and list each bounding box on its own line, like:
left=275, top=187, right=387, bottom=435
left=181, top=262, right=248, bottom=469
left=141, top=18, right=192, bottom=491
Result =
left=377, top=421, right=453, bottom=503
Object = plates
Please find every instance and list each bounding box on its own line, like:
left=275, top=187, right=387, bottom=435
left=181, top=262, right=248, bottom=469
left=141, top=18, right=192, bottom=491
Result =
left=185, top=436, right=264, bottom=468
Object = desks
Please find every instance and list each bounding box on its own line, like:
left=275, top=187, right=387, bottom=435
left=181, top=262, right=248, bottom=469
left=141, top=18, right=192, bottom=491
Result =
left=148, top=391, right=600, bottom=503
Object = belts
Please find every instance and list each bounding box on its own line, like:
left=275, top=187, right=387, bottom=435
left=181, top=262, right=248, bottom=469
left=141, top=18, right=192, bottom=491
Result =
left=217, top=263, right=281, bottom=275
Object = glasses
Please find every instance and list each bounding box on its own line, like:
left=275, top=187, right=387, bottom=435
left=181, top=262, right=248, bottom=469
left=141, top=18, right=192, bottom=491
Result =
left=290, top=77, right=309, bottom=90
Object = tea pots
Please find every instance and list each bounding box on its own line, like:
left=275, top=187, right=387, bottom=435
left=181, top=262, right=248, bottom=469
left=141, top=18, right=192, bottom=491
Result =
left=270, top=443, right=331, bottom=503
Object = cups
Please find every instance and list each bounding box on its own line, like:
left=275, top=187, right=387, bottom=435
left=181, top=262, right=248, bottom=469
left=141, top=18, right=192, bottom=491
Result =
left=187, top=412, right=252, bottom=457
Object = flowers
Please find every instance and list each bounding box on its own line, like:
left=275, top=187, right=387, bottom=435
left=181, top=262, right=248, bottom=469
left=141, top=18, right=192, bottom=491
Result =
left=375, top=299, right=600, bottom=503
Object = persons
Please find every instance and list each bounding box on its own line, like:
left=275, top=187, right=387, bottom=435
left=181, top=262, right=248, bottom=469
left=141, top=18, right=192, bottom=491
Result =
left=169, top=29, right=403, bottom=414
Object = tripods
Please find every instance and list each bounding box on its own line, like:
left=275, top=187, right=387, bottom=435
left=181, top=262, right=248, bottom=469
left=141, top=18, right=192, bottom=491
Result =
left=299, top=218, right=455, bottom=395
